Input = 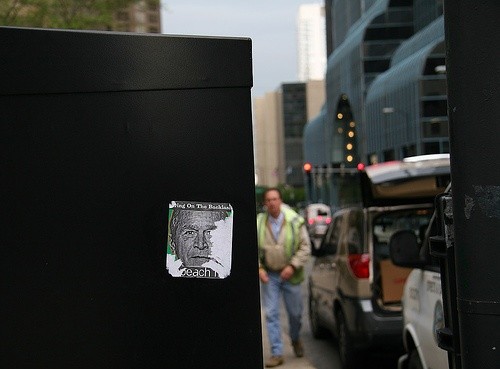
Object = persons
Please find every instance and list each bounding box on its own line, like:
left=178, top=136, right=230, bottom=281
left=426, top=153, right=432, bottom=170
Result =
left=170, top=208, right=231, bottom=267
left=256, top=187, right=312, bottom=368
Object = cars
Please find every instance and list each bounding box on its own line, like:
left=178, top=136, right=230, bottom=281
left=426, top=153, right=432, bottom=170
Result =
left=308, top=154, right=450, bottom=369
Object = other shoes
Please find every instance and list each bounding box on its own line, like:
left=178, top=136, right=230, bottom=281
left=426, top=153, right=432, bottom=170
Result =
left=293, top=340, right=305, bottom=356
left=266, top=355, right=283, bottom=366
